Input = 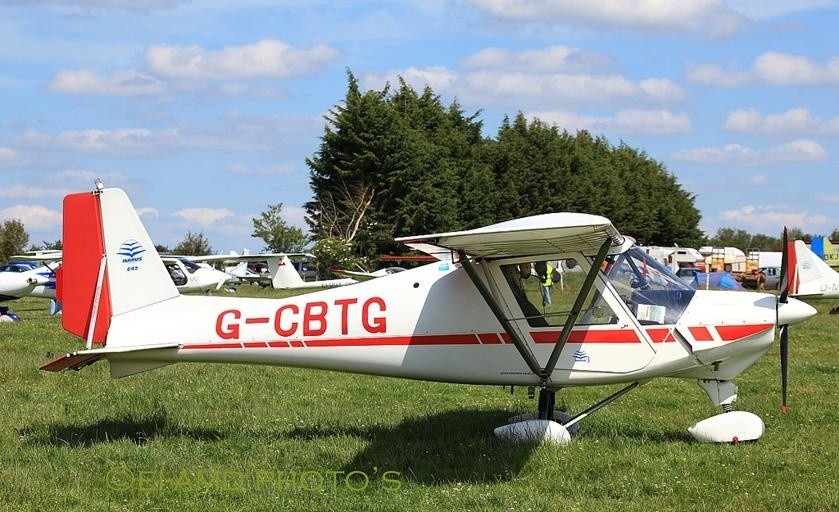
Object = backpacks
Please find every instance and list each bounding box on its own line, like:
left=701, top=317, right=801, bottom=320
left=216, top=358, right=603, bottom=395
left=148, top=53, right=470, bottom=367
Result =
left=552, top=268, right=560, bottom=283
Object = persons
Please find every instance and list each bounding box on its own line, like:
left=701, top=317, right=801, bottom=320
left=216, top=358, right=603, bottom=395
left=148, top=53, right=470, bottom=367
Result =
left=538, top=263, right=554, bottom=306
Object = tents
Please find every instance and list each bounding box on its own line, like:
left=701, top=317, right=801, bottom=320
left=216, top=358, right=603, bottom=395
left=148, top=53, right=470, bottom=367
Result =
left=687, top=270, right=745, bottom=289
